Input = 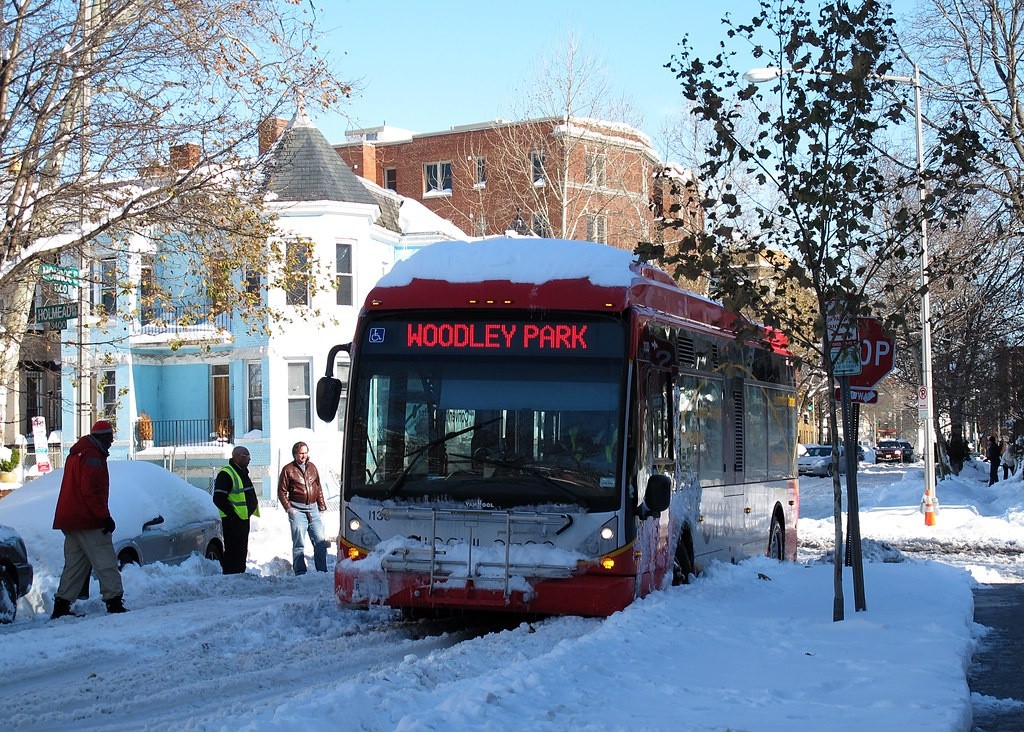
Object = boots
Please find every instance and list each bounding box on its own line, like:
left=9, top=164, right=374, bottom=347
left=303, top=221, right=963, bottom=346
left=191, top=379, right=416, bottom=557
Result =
left=49, top=597, right=85, bottom=619
left=102, top=595, right=130, bottom=613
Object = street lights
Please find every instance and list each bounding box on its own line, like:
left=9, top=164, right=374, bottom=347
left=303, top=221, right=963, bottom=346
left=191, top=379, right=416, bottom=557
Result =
left=742, top=63, right=941, bottom=515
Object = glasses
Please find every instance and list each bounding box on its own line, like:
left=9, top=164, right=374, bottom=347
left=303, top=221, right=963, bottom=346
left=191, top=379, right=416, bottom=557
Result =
left=239, top=453, right=251, bottom=459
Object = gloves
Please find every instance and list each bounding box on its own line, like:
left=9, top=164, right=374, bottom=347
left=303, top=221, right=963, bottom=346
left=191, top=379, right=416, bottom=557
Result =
left=99, top=516, right=116, bottom=535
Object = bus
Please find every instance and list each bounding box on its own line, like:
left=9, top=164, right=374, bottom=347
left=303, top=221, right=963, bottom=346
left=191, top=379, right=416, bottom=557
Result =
left=316, top=238, right=799, bottom=617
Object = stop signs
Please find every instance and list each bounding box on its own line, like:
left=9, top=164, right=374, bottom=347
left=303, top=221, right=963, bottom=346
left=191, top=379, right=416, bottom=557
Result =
left=821, top=317, right=895, bottom=388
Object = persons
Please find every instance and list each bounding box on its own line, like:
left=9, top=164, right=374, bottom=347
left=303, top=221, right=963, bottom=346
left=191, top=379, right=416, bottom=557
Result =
left=212, top=446, right=260, bottom=575
left=52, top=420, right=131, bottom=618
left=1001, top=446, right=1015, bottom=480
left=983, top=436, right=1000, bottom=487
left=277, top=442, right=327, bottom=576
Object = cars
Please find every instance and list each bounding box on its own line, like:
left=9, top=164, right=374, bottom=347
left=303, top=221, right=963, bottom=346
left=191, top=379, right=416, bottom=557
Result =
left=873, top=440, right=905, bottom=465
left=0, top=460, right=226, bottom=580
left=843, top=441, right=866, bottom=461
left=0, top=524, right=34, bottom=625
left=798, top=446, right=859, bottom=478
left=970, top=452, right=985, bottom=461
left=898, top=441, right=914, bottom=458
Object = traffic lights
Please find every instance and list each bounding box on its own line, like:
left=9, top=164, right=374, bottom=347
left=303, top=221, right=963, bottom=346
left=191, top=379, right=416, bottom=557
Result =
left=807, top=401, right=813, bottom=411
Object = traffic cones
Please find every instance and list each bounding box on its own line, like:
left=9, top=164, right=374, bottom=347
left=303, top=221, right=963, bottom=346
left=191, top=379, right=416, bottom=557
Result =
left=925, top=489, right=936, bottom=526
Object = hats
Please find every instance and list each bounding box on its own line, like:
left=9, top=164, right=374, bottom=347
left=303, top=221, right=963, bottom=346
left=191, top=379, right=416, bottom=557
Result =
left=91, top=421, right=113, bottom=434
left=989, top=436, right=995, bottom=443
